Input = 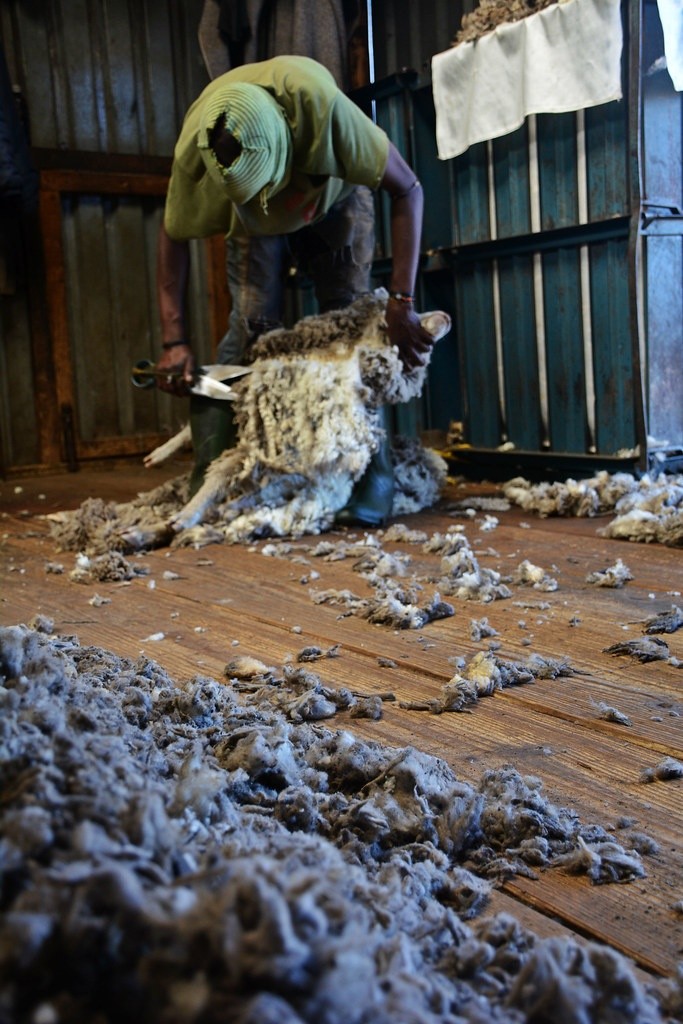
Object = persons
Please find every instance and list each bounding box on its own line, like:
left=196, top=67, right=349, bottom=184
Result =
left=153, top=55, right=435, bottom=531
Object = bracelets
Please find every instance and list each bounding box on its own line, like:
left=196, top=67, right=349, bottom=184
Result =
left=388, top=290, right=415, bottom=304
left=161, top=338, right=190, bottom=351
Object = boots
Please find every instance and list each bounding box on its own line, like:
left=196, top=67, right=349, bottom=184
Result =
left=186, top=390, right=238, bottom=503
left=336, top=400, right=396, bottom=524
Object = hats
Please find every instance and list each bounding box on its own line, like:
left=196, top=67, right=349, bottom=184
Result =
left=197, top=80, right=287, bottom=206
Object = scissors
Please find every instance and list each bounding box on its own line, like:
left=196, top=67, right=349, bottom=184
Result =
left=130, top=357, right=250, bottom=402
